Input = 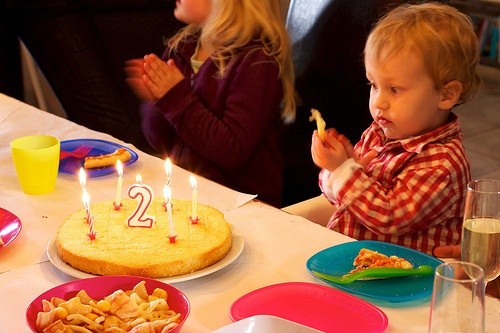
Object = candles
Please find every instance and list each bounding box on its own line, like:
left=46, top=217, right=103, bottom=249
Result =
left=78, top=167, right=87, bottom=194
left=189, top=176, right=200, bottom=224
left=114, top=159, right=123, bottom=210
left=82, top=192, right=97, bottom=239
left=127, top=175, right=155, bottom=228
left=163, top=186, right=177, bottom=243
left=163, top=157, right=172, bottom=186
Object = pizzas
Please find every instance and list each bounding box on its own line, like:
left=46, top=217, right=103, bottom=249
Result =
left=342, top=248, right=413, bottom=281
left=84, top=148, right=131, bottom=169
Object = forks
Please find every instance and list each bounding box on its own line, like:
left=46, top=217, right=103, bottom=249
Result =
left=59, top=144, right=94, bottom=162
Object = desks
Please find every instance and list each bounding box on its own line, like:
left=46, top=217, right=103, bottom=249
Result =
left=0, top=92, right=500, bottom=333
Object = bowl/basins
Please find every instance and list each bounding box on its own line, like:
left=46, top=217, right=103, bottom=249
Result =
left=24, top=275, right=191, bottom=333
left=206, top=315, right=326, bottom=333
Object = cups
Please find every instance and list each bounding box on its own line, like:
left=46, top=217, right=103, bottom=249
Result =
left=428, top=261, right=486, bottom=333
left=10, top=134, right=60, bottom=195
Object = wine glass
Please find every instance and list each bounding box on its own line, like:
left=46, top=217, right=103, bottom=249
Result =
left=458, top=178, right=500, bottom=333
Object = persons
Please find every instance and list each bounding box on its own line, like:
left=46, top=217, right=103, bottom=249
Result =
left=125, top=0, right=297, bottom=209
left=310, top=3, right=481, bottom=259
left=281, top=0, right=392, bottom=209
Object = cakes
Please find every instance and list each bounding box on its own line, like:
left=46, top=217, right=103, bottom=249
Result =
left=57, top=197, right=232, bottom=278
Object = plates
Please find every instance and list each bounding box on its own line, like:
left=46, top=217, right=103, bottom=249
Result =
left=228, top=281, right=388, bottom=333
left=305, top=240, right=455, bottom=303
left=47, top=216, right=245, bottom=284
left=58, top=138, right=139, bottom=179
left=0, top=207, right=22, bottom=252
